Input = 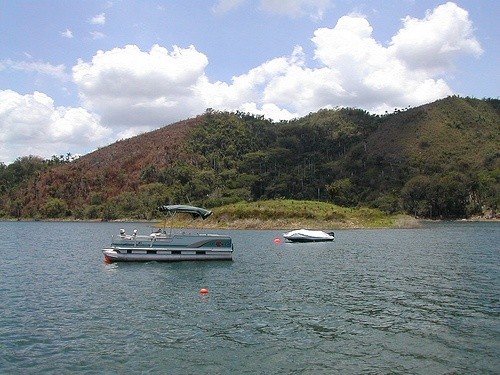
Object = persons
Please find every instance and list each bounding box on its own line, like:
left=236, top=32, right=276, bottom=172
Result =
left=133, top=228, right=138, bottom=236
left=157, top=227, right=162, bottom=233
left=120, top=228, right=126, bottom=235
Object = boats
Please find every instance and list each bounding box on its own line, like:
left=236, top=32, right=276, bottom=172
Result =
left=102, top=205, right=233, bottom=260
left=283, top=228, right=335, bottom=241
